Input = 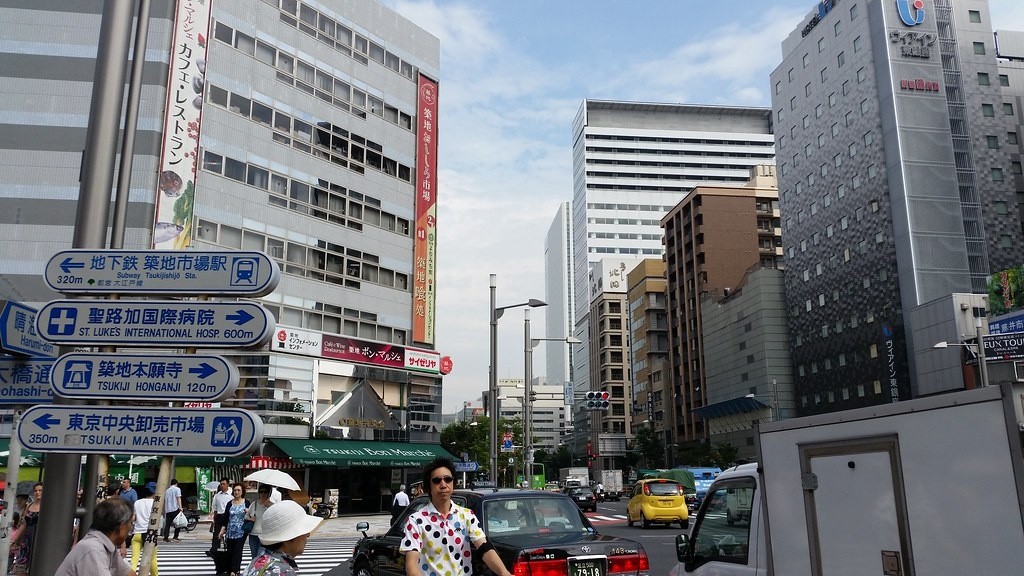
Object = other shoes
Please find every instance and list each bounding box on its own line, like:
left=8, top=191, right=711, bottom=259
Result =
left=235, top=572, right=242, bottom=576
left=173, top=536, right=181, bottom=542
left=163, top=538, right=171, bottom=544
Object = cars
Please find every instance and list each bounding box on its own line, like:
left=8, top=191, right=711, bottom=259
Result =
left=545, top=478, right=598, bottom=513
left=349, top=488, right=650, bottom=576
left=627, top=478, right=689, bottom=528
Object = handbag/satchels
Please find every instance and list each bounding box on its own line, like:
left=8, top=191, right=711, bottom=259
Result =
left=242, top=501, right=257, bottom=533
left=173, top=511, right=188, bottom=529
left=211, top=536, right=232, bottom=576
left=600, top=489, right=604, bottom=492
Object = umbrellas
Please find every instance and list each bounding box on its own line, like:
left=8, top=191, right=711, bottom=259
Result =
left=243, top=469, right=301, bottom=522
left=16, top=480, right=37, bottom=494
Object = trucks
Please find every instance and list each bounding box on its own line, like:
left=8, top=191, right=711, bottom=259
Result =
left=667, top=382, right=1023, bottom=576
left=560, top=467, right=624, bottom=502
left=638, top=467, right=754, bottom=526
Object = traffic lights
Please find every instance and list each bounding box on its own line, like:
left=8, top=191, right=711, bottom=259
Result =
left=585, top=392, right=610, bottom=410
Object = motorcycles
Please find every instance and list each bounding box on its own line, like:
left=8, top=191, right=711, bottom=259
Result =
left=592, top=489, right=605, bottom=503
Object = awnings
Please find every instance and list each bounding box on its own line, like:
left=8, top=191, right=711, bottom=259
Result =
left=0, top=439, right=252, bottom=467
left=270, top=438, right=461, bottom=467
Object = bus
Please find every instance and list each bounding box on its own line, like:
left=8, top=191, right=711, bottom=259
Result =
left=507, top=463, right=545, bottom=490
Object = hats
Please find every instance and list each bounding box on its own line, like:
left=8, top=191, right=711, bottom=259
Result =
left=145, top=481, right=156, bottom=496
left=257, top=499, right=324, bottom=545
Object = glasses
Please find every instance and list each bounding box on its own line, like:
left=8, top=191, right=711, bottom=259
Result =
left=429, top=477, right=454, bottom=484
left=259, top=490, right=268, bottom=494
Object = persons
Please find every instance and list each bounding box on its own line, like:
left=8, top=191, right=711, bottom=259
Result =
left=366, top=477, right=378, bottom=512
left=7, top=483, right=83, bottom=576
left=393, top=485, right=410, bottom=517
left=594, top=481, right=603, bottom=503
left=217, top=482, right=254, bottom=576
left=99, top=479, right=127, bottom=558
left=414, top=484, right=423, bottom=498
left=398, top=456, right=515, bottom=576
left=244, top=484, right=274, bottom=560
left=53, top=498, right=138, bottom=576
left=204, top=477, right=234, bottom=557
left=131, top=482, right=163, bottom=576
left=119, top=478, right=137, bottom=548
left=240, top=499, right=324, bottom=576
left=162, top=479, right=183, bottom=543
left=269, top=485, right=282, bottom=504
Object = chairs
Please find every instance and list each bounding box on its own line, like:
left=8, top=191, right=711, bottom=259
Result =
left=492, top=507, right=520, bottom=527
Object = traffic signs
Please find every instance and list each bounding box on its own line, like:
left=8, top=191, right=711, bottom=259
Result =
left=17, top=353, right=264, bottom=457
left=35, top=302, right=276, bottom=349
left=453, top=462, right=479, bottom=472
left=0, top=300, right=59, bottom=405
left=45, top=248, right=281, bottom=297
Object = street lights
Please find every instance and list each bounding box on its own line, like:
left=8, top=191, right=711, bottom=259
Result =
left=489, top=274, right=549, bottom=483
left=744, top=378, right=780, bottom=421
left=497, top=309, right=582, bottom=486
left=642, top=410, right=680, bottom=469
left=931, top=317, right=989, bottom=387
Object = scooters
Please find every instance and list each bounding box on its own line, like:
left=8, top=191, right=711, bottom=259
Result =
left=184, top=510, right=200, bottom=531
